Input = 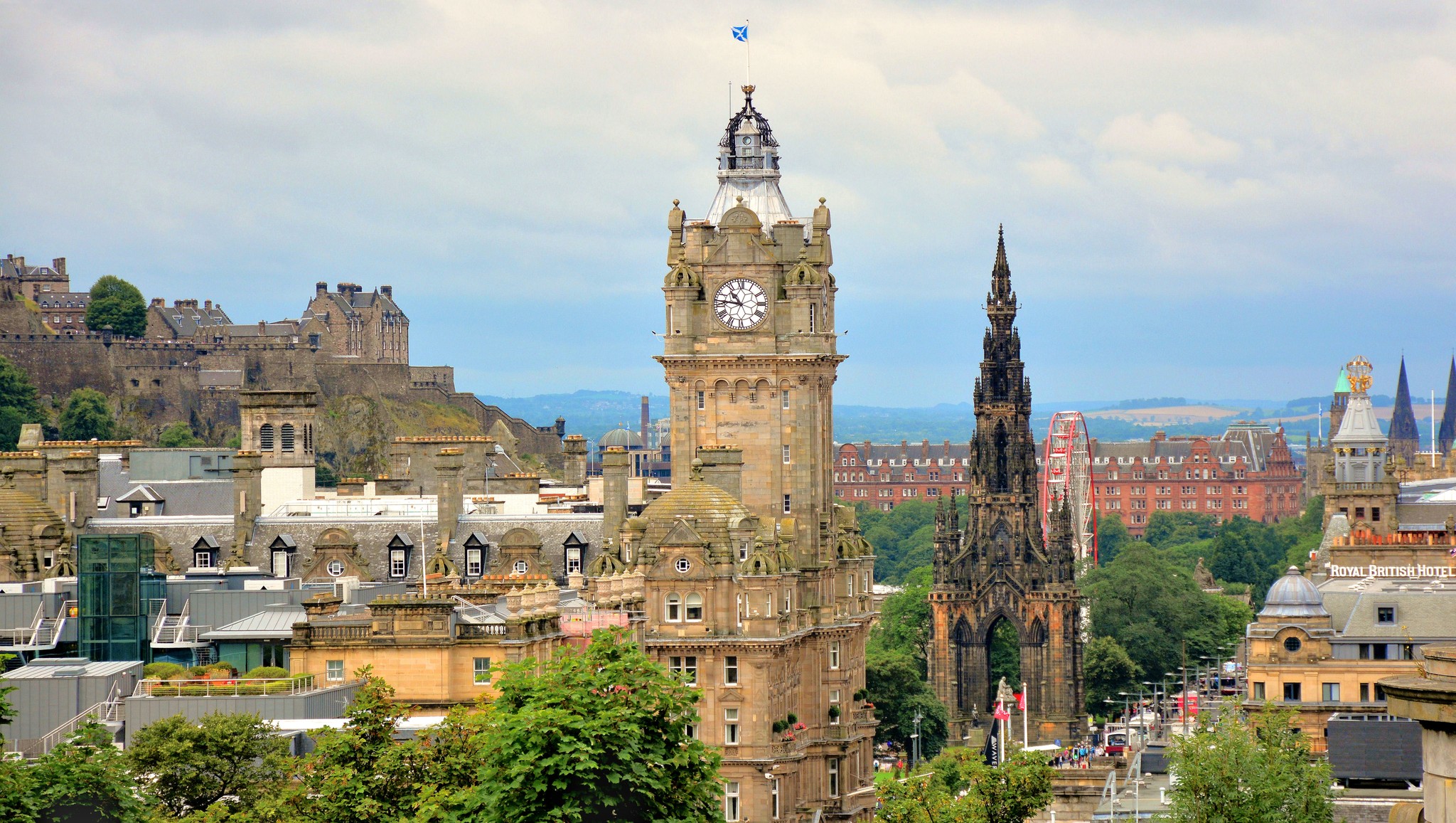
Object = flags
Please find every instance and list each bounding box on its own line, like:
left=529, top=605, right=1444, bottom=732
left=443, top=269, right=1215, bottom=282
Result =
left=994, top=702, right=1010, bottom=721
left=1319, top=408, right=1323, bottom=416
left=1013, top=692, right=1025, bottom=711
left=1449, top=548, right=1456, bottom=558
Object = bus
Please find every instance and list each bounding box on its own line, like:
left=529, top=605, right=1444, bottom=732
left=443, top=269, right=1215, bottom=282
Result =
left=1128, top=711, right=1162, bottom=738
left=1105, top=729, right=1139, bottom=756
left=1220, top=674, right=1245, bottom=695
left=1128, top=712, right=1163, bottom=742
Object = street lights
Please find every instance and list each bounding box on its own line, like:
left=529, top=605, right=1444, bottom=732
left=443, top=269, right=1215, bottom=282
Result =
left=1103, top=638, right=1253, bottom=754
left=910, top=704, right=924, bottom=771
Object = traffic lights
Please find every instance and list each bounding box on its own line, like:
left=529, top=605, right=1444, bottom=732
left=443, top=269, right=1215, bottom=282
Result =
left=1073, top=748, right=1078, bottom=759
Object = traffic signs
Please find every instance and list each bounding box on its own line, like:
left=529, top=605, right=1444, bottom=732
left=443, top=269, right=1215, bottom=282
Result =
left=1090, top=727, right=1098, bottom=731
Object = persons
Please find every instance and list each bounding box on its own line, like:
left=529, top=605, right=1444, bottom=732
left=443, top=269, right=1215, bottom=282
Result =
left=1137, top=697, right=1178, bottom=723
left=996, top=677, right=1017, bottom=701
left=1064, top=741, right=1105, bottom=769
left=1445, top=514, right=1455, bottom=536
left=1118, top=737, right=1123, bottom=743
left=1113, top=736, right=1118, bottom=743
left=874, top=762, right=878, bottom=773
left=1048, top=754, right=1065, bottom=769
left=980, top=748, right=984, bottom=756
left=970, top=707, right=981, bottom=729
left=1389, top=516, right=1400, bottom=530
left=898, top=759, right=903, bottom=773
left=1194, top=558, right=1219, bottom=589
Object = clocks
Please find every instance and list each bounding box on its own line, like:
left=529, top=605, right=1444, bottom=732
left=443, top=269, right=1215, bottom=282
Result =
left=714, top=278, right=768, bottom=330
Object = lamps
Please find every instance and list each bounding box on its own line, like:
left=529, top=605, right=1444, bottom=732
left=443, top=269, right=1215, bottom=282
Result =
left=861, top=783, right=865, bottom=786
left=755, top=765, right=780, bottom=772
left=818, top=329, right=835, bottom=334
left=834, top=801, right=838, bottom=806
left=773, top=238, right=777, bottom=243
left=859, top=591, right=872, bottom=601
left=650, top=628, right=663, bottom=633
left=777, top=616, right=783, bottom=620
left=740, top=816, right=749, bottom=823
left=798, top=329, right=810, bottom=334
left=651, top=330, right=666, bottom=336
left=675, top=329, right=681, bottom=336
left=834, top=613, right=847, bottom=619
left=799, top=815, right=803, bottom=820
left=785, top=613, right=789, bottom=619
left=805, top=239, right=810, bottom=243
left=834, top=330, right=848, bottom=335
left=705, top=240, right=709, bottom=243
left=702, top=628, right=709, bottom=632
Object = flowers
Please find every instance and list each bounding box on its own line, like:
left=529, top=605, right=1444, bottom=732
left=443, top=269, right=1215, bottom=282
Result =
left=864, top=703, right=875, bottom=709
left=784, top=723, right=806, bottom=741
